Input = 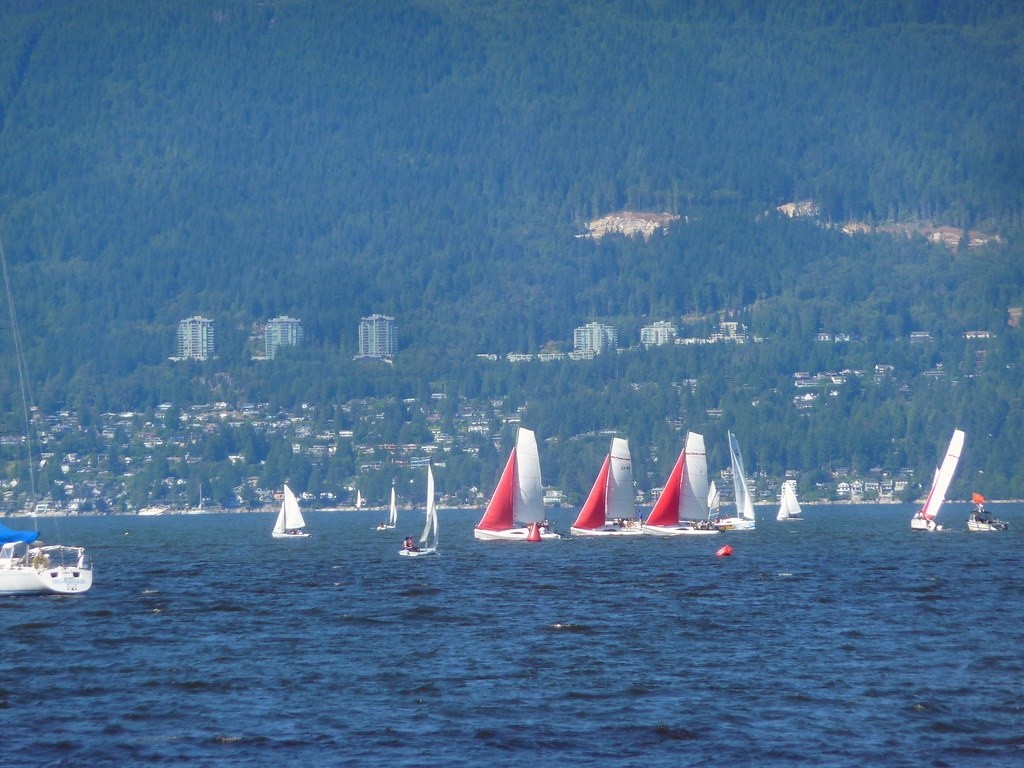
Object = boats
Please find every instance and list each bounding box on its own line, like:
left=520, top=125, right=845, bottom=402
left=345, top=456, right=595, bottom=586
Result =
left=967, top=492, right=1009, bottom=531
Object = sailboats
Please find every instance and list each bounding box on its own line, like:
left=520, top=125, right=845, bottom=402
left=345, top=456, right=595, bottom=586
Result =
left=570, top=437, right=643, bottom=537
left=376, top=486, right=398, bottom=530
left=910, top=428, right=966, bottom=531
left=272, top=483, right=311, bottom=538
left=709, top=430, right=755, bottom=531
left=356, top=489, right=361, bottom=511
left=397, top=463, right=439, bottom=557
left=474, top=426, right=562, bottom=541
left=643, top=429, right=720, bottom=536
left=776, top=480, right=804, bottom=521
left=0, top=238, right=93, bottom=595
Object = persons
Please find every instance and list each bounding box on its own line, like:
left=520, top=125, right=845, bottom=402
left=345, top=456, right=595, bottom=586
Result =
left=380, top=523, right=384, bottom=528
left=793, top=514, right=797, bottom=518
left=619, top=518, right=637, bottom=528
left=637, top=509, right=644, bottom=528
left=404, top=535, right=420, bottom=552
left=914, top=511, right=925, bottom=519
left=13, top=553, right=21, bottom=558
left=527, top=519, right=550, bottom=532
left=291, top=528, right=304, bottom=535
left=690, top=519, right=715, bottom=530
left=35, top=554, right=51, bottom=574
